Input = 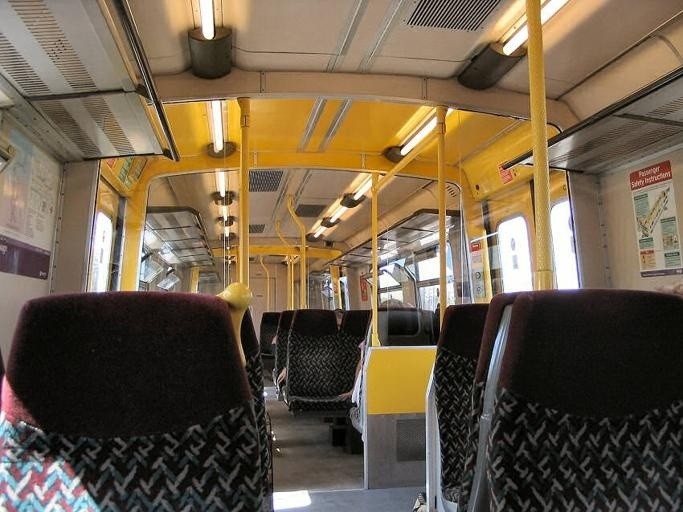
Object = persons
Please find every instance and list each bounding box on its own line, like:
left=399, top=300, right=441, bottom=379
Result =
left=339, top=299, right=421, bottom=410
left=334, top=307, right=345, bottom=328
left=277, top=364, right=288, bottom=385
left=271, top=334, right=277, bottom=356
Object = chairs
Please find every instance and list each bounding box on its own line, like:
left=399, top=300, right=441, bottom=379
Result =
left=0, top=292, right=274, bottom=511
left=258, top=309, right=440, bottom=455
left=231, top=304, right=274, bottom=498
left=412, top=286, right=683, bottom=512
